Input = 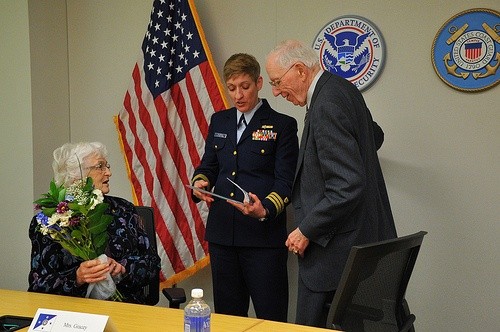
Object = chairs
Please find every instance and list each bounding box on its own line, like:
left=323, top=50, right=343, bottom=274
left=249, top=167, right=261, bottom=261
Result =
left=326, top=230, right=428, bottom=332
left=134, top=206, right=186, bottom=308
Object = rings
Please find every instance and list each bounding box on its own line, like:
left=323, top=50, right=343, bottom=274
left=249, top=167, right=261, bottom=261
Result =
left=241, top=208, right=246, bottom=211
left=113, top=262, right=117, bottom=267
left=294, top=251, right=299, bottom=255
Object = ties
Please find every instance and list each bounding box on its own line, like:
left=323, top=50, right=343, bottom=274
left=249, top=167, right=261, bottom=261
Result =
left=304, top=105, right=309, bottom=127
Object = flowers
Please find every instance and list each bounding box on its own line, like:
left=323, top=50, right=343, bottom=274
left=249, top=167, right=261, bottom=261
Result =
left=33, top=152, right=125, bottom=301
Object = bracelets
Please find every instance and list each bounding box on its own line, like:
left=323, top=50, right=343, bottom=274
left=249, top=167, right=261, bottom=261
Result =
left=258, top=208, right=269, bottom=222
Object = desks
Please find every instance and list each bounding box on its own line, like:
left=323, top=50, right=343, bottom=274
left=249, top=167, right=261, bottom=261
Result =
left=0, top=287, right=346, bottom=332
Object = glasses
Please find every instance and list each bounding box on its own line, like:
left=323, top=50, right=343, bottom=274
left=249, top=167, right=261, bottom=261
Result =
left=269, top=61, right=306, bottom=88
left=83, top=163, right=110, bottom=171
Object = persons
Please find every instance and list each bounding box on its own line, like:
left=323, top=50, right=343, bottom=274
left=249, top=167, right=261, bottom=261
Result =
left=28, top=143, right=162, bottom=304
left=190, top=53, right=299, bottom=323
left=264, top=40, right=398, bottom=329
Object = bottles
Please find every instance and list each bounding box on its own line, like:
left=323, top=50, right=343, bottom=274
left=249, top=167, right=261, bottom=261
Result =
left=183, top=288, right=211, bottom=332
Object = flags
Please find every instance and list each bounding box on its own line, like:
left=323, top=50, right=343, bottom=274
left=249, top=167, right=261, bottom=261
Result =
left=111, top=0, right=231, bottom=291
